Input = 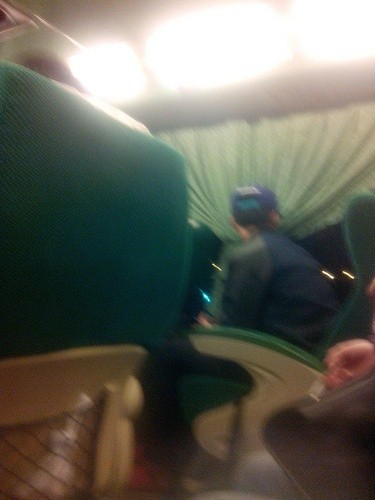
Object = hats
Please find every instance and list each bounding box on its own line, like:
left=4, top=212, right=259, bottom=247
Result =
left=229, top=184, right=283, bottom=218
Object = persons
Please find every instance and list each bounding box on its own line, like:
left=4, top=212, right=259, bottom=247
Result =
left=120, top=183, right=341, bottom=494
left=189, top=276, right=375, bottom=500
